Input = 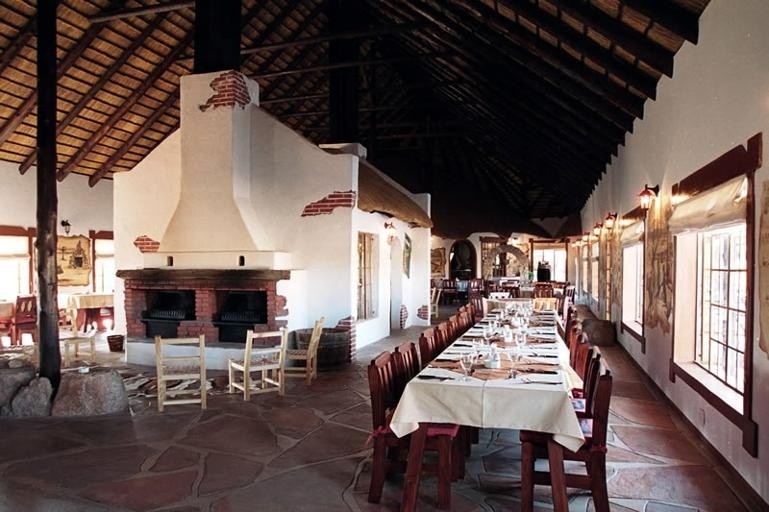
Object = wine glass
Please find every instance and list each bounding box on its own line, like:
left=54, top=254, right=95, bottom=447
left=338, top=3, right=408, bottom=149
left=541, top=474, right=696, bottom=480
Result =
left=460, top=300, right=533, bottom=382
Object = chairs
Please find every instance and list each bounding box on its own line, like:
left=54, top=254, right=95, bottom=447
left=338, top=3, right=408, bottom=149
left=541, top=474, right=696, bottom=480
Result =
left=228, top=328, right=287, bottom=402
left=58, top=310, right=95, bottom=367
left=155, top=335, right=207, bottom=413
left=272, top=317, right=323, bottom=387
left=8, top=295, right=39, bottom=348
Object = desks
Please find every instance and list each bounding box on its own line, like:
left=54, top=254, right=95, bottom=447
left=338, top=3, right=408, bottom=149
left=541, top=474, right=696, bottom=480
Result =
left=0, top=298, right=14, bottom=339
left=56, top=292, right=116, bottom=333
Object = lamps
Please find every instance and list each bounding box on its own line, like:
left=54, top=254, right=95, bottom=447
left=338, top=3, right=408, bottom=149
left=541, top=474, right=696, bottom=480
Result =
left=604, top=211, right=617, bottom=229
left=593, top=223, right=603, bottom=237
left=581, top=231, right=590, bottom=243
left=639, top=184, right=659, bottom=210
